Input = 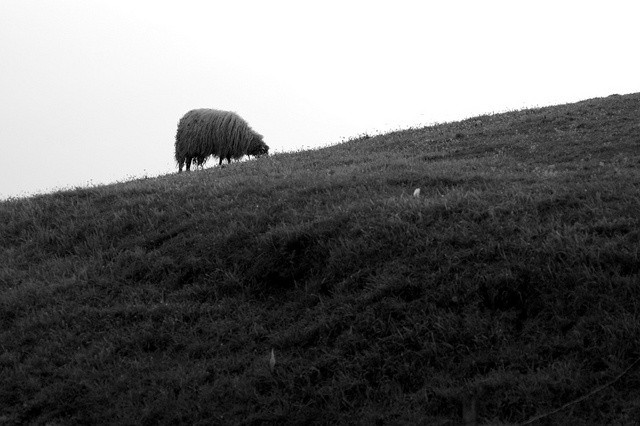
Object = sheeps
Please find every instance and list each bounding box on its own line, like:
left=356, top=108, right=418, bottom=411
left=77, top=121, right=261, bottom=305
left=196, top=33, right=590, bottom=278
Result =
left=173, top=107, right=270, bottom=173
